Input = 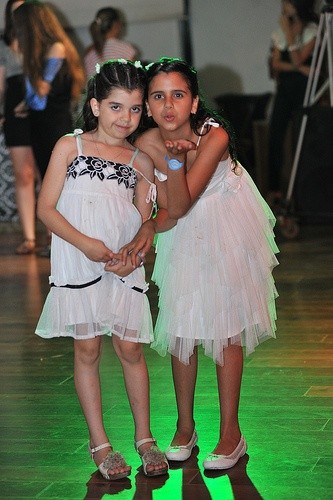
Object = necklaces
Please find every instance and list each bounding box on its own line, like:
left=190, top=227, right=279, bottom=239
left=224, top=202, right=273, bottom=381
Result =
left=92, top=131, right=126, bottom=160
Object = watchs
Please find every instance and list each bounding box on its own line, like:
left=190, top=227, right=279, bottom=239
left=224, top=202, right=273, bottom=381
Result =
left=137, top=251, right=146, bottom=267
left=165, top=156, right=185, bottom=170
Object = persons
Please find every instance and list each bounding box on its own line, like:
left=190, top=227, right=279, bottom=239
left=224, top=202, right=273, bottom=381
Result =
left=132, top=57, right=280, bottom=470
left=0, top=0, right=84, bottom=257
left=264, top=0, right=318, bottom=208
left=35, top=58, right=169, bottom=480
left=84, top=6, right=137, bottom=82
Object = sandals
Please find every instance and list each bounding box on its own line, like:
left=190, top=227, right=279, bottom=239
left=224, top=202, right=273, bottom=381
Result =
left=134, top=437, right=169, bottom=477
left=90, top=443, right=132, bottom=481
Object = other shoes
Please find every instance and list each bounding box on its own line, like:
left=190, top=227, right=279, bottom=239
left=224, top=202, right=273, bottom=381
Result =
left=16, top=240, right=35, bottom=254
left=203, top=433, right=248, bottom=469
left=164, top=430, right=198, bottom=461
